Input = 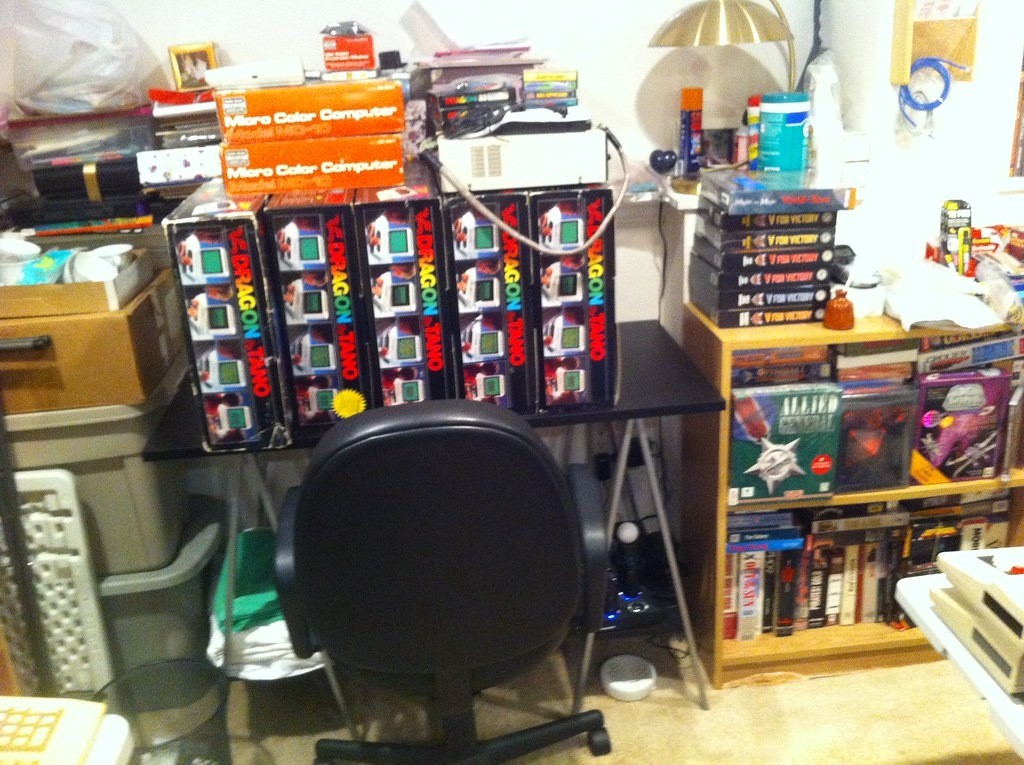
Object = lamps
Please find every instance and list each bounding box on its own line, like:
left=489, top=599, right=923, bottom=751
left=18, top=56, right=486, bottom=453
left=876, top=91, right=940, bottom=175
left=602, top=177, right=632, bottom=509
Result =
left=648, top=0, right=797, bottom=93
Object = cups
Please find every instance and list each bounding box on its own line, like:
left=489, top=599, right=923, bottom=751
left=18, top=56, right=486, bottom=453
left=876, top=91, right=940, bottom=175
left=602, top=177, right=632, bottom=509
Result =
left=0, top=238, right=132, bottom=285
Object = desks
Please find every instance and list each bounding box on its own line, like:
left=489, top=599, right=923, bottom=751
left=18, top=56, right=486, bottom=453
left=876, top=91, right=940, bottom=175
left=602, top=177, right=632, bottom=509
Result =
left=141, top=320, right=727, bottom=740
left=895, top=573, right=1024, bottom=760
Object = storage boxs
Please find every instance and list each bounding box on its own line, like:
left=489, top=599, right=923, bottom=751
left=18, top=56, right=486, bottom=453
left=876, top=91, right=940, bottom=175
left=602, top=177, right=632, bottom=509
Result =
left=99, top=496, right=223, bottom=715
left=213, top=80, right=406, bottom=145
left=435, top=175, right=535, bottom=417
left=221, top=133, right=406, bottom=197
left=0, top=248, right=153, bottom=319
left=426, top=61, right=579, bottom=130
left=688, top=170, right=1024, bottom=507
left=0, top=267, right=185, bottom=415
left=160, top=180, right=294, bottom=455
left=529, top=183, right=618, bottom=415
left=437, top=128, right=607, bottom=194
left=260, top=188, right=378, bottom=435
left=354, top=160, right=456, bottom=412
left=7, top=106, right=160, bottom=173
left=0, top=345, right=195, bottom=578
left=136, top=145, right=223, bottom=189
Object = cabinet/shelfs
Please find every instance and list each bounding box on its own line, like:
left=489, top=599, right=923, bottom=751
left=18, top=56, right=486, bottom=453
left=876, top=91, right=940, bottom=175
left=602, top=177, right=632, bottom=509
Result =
left=680, top=301, right=1024, bottom=689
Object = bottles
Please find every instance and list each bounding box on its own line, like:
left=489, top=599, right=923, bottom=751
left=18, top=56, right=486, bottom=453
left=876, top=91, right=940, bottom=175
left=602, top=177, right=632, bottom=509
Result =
left=733, top=112, right=748, bottom=170
left=679, top=87, right=702, bottom=182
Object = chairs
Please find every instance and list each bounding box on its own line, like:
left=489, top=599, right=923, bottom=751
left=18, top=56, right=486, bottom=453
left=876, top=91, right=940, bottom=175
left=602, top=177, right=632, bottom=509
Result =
left=271, top=400, right=611, bottom=765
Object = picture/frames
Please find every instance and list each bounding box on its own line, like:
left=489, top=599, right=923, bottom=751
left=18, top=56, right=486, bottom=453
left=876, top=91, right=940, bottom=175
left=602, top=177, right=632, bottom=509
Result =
left=166, top=43, right=219, bottom=92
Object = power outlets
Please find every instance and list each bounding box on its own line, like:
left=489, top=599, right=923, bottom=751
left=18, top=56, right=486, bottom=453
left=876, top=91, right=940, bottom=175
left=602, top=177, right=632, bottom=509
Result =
left=592, top=427, right=657, bottom=489
